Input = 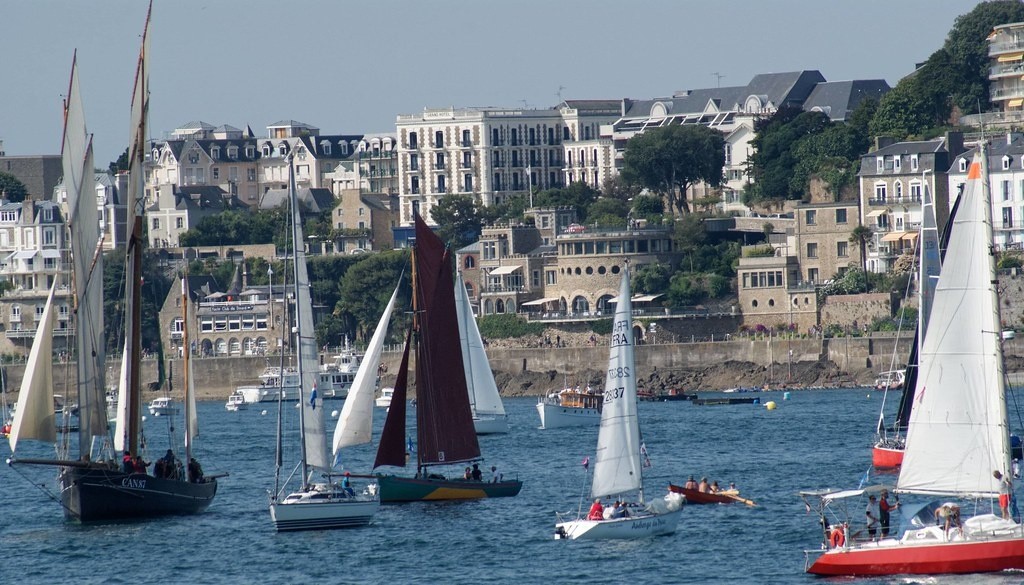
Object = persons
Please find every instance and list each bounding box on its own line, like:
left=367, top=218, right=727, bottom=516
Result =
left=993, top=471, right=1012, bottom=519
left=574, top=383, right=603, bottom=394
left=879, top=488, right=901, bottom=540
left=685, top=474, right=737, bottom=493
left=154, top=449, right=186, bottom=482
left=189, top=457, right=203, bottom=484
left=865, top=494, right=880, bottom=541
left=464, top=463, right=483, bottom=481
left=123, top=450, right=152, bottom=475
left=491, top=466, right=504, bottom=483
left=342, top=471, right=354, bottom=497
left=935, top=502, right=962, bottom=541
left=586, top=497, right=629, bottom=521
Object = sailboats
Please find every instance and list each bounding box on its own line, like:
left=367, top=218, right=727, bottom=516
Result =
left=801, top=101, right=1024, bottom=576
left=872, top=168, right=1024, bottom=469
left=268, top=153, right=524, bottom=532
left=6, top=0, right=230, bottom=524
left=553, top=257, right=684, bottom=541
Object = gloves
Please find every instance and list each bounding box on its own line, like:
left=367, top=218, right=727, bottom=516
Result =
left=936, top=519, right=939, bottom=525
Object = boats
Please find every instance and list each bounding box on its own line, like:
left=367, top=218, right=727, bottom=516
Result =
left=0, top=367, right=118, bottom=437
left=536, top=370, right=605, bottom=429
left=376, top=388, right=395, bottom=407
left=636, top=387, right=698, bottom=402
left=148, top=397, right=180, bottom=416
left=225, top=391, right=249, bottom=411
left=668, top=480, right=736, bottom=504
left=235, top=334, right=364, bottom=403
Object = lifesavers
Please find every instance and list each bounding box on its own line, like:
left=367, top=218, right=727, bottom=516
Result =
left=830, top=528, right=844, bottom=548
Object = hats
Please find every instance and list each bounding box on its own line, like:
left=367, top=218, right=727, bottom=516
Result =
left=345, top=472, right=350, bottom=477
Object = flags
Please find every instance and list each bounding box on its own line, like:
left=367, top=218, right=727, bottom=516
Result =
left=582, top=455, right=590, bottom=471
left=310, top=379, right=317, bottom=411
